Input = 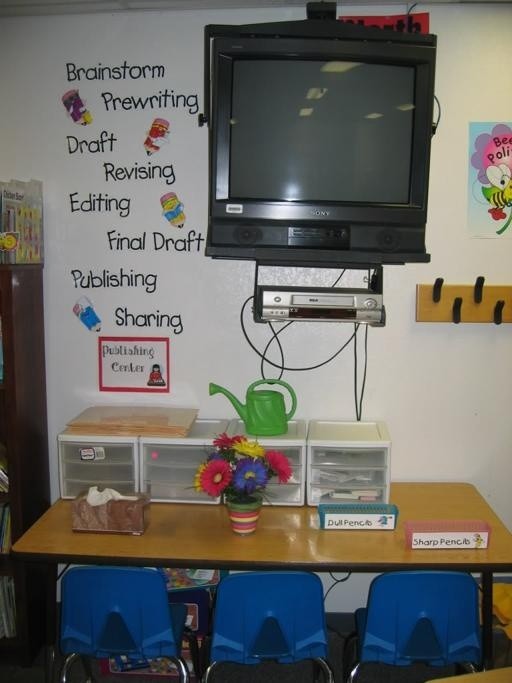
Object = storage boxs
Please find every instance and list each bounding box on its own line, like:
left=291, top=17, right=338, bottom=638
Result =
left=137, top=423, right=225, bottom=506
left=71, top=499, right=147, bottom=535
left=57, top=434, right=139, bottom=501
left=307, top=421, right=392, bottom=508
left=223, top=421, right=304, bottom=507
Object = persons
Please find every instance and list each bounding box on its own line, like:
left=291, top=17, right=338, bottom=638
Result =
left=151, top=364, right=162, bottom=380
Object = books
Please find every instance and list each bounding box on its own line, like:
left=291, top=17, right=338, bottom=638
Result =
left=0, top=447, right=11, bottom=552
left=0, top=576, right=16, bottom=639
left=332, top=489, right=381, bottom=501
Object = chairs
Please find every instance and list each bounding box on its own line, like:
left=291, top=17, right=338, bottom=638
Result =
left=346, top=570, right=480, bottom=682
left=205, top=570, right=334, bottom=683
left=55, top=566, right=188, bottom=683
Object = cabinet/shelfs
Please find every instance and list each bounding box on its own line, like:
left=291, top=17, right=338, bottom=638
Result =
left=0, top=263, right=50, bottom=546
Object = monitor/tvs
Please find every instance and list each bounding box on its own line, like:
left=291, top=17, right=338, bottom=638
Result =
left=210, top=34, right=437, bottom=226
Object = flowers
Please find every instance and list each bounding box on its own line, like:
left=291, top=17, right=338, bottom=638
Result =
left=193, top=430, right=292, bottom=500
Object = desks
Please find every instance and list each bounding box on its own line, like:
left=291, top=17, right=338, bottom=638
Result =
left=11, top=480, right=512, bottom=675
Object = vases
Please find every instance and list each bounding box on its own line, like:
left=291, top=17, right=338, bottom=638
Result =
left=226, top=496, right=263, bottom=536
